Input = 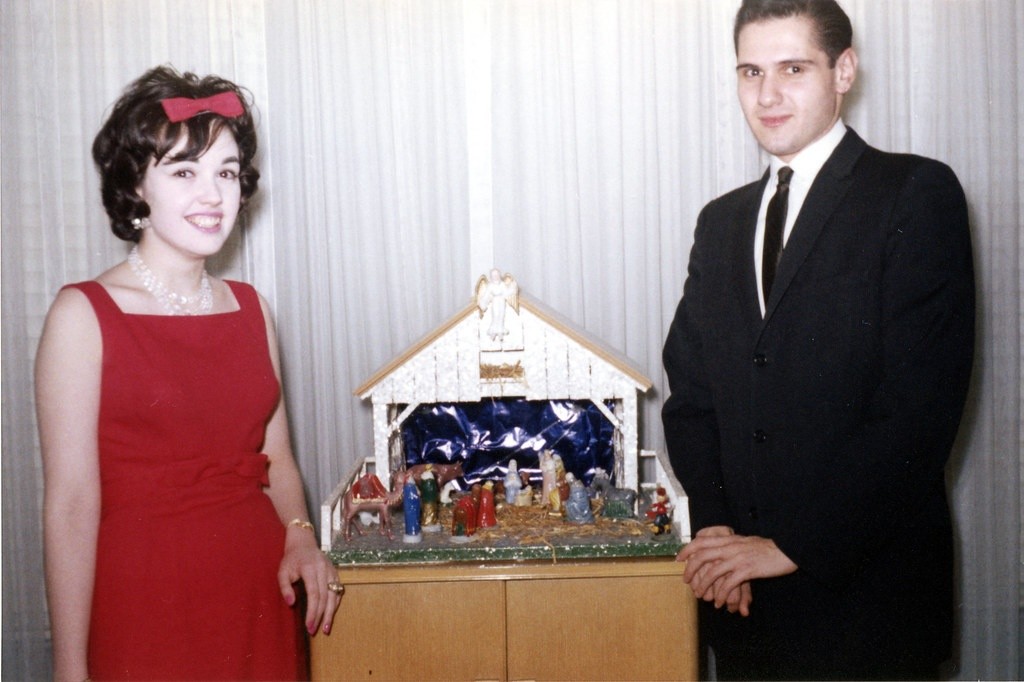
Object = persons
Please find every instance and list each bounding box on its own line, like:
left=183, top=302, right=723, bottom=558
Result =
left=420, top=470, right=438, bottom=525
left=504, top=460, right=523, bottom=503
left=488, top=269, right=510, bottom=341
left=538, top=449, right=592, bottom=523
left=33, top=66, right=342, bottom=682
left=663, top=0, right=976, bottom=682
left=645, top=482, right=672, bottom=534
left=402, top=470, right=422, bottom=542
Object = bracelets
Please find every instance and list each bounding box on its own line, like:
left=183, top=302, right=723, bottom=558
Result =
left=287, top=519, right=314, bottom=532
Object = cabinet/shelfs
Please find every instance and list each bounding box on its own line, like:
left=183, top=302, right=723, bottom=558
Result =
left=308, top=556, right=698, bottom=682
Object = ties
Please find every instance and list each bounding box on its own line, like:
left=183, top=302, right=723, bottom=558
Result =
left=762, top=166, right=794, bottom=310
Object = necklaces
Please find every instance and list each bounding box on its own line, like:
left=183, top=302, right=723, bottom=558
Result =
left=127, top=246, right=212, bottom=316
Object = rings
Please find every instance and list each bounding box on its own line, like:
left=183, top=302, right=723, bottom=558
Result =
left=328, top=582, right=344, bottom=592
